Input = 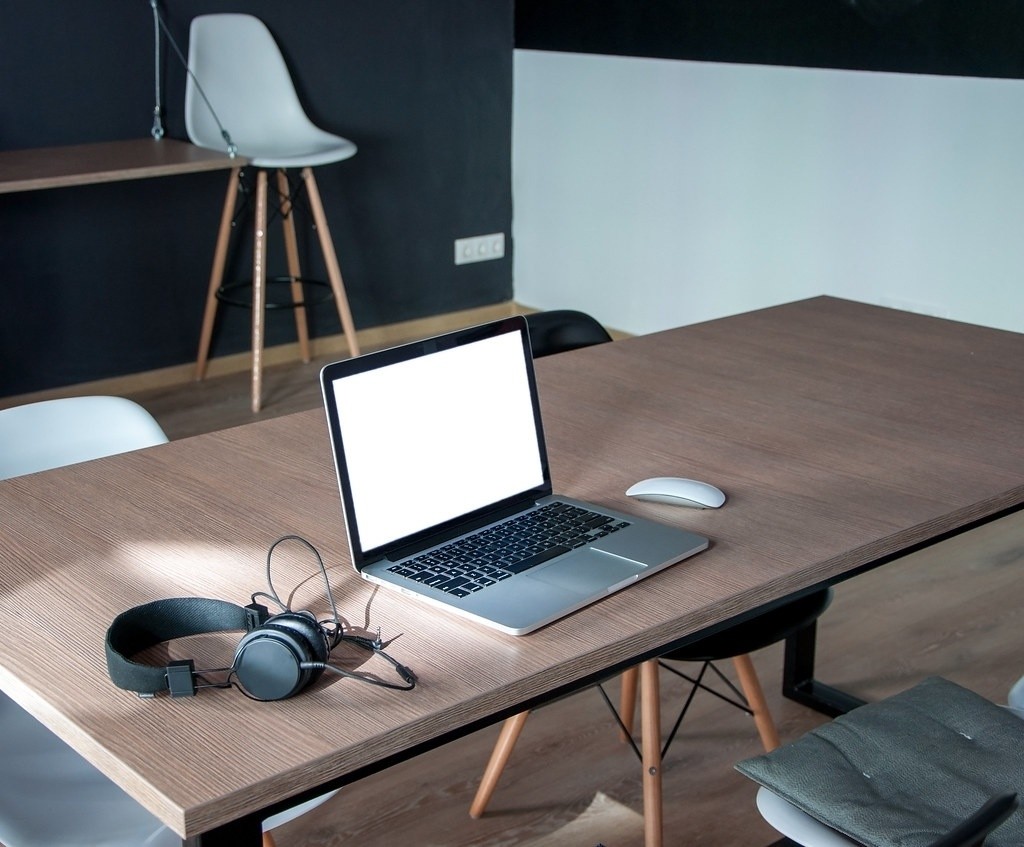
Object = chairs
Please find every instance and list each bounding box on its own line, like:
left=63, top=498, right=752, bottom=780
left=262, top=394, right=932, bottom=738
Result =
left=732, top=674, right=1024, bottom=847
left=185, top=11, right=365, bottom=412
left=0, top=394, right=344, bottom=847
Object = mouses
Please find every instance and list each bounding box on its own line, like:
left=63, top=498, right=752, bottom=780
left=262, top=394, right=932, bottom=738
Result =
left=626, top=477, right=726, bottom=509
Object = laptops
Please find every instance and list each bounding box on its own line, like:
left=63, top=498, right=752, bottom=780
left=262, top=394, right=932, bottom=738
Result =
left=320, top=315, right=710, bottom=637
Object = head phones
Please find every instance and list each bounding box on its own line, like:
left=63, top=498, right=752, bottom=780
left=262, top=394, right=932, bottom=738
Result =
left=105, top=598, right=330, bottom=702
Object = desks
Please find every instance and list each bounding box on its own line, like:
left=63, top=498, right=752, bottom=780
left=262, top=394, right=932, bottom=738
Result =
left=0, top=294, right=1024, bottom=847
